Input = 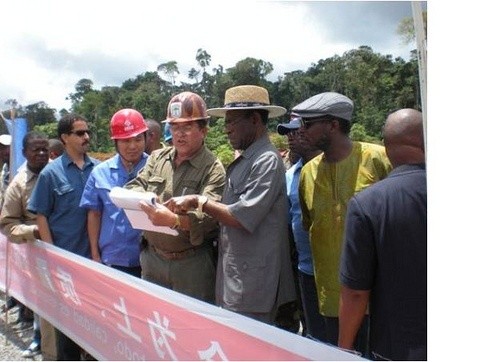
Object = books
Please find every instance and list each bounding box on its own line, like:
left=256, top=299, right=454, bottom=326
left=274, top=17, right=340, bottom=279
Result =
left=109, top=185, right=181, bottom=237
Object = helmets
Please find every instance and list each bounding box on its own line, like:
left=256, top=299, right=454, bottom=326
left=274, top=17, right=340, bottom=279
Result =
left=110, top=108, right=149, bottom=140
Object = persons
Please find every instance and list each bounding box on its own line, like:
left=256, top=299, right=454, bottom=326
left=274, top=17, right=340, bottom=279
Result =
left=0, top=130, right=53, bottom=357
left=77, top=108, right=153, bottom=280
left=143, top=118, right=166, bottom=156
left=335, top=108, right=428, bottom=361
left=0, top=133, right=17, bottom=314
left=11, top=158, right=53, bottom=335
left=162, top=85, right=298, bottom=327
left=48, top=138, right=65, bottom=164
left=289, top=92, right=396, bottom=357
left=277, top=118, right=341, bottom=346
left=25, top=113, right=105, bottom=260
left=122, top=91, right=226, bottom=306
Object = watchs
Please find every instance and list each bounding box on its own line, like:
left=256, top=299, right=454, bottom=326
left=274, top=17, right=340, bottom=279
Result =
left=197, top=195, right=208, bottom=212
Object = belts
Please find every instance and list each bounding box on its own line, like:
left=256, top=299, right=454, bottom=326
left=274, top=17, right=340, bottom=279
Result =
left=154, top=245, right=207, bottom=260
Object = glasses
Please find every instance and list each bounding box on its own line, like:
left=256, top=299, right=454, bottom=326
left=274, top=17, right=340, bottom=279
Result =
left=222, top=111, right=248, bottom=132
left=169, top=122, right=205, bottom=135
left=303, top=118, right=330, bottom=130
left=286, top=131, right=302, bottom=138
left=66, top=130, right=91, bottom=135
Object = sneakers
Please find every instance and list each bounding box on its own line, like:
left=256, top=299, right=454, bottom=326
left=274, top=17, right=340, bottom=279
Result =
left=20, top=339, right=40, bottom=357
left=10, top=321, right=33, bottom=333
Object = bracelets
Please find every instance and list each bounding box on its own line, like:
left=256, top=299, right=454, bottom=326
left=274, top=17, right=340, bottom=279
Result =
left=170, top=214, right=180, bottom=231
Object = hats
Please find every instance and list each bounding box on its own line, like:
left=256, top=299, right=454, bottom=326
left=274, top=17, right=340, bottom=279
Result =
left=204, top=84, right=287, bottom=118
left=276, top=117, right=302, bottom=135
left=291, top=91, right=354, bottom=123
left=158, top=92, right=210, bottom=124
